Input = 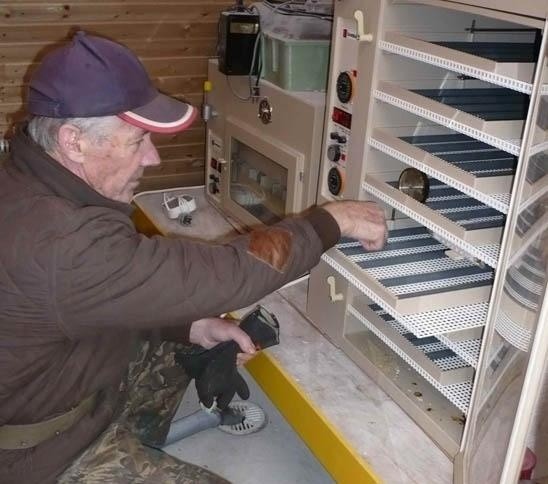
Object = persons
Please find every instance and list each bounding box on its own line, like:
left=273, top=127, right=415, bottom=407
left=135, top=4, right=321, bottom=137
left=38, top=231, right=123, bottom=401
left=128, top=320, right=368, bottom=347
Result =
left=0, top=31, right=390, bottom=484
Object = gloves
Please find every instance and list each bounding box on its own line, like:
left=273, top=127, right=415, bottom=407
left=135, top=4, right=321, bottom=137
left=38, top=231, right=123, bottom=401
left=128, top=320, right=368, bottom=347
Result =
left=184, top=306, right=279, bottom=410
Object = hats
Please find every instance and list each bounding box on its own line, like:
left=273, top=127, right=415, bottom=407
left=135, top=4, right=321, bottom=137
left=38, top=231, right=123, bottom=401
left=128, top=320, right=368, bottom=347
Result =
left=24, top=31, right=198, bottom=133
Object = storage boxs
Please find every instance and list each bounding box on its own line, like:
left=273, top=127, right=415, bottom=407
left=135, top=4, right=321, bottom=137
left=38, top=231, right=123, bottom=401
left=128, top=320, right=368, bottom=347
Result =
left=261, top=29, right=332, bottom=92
